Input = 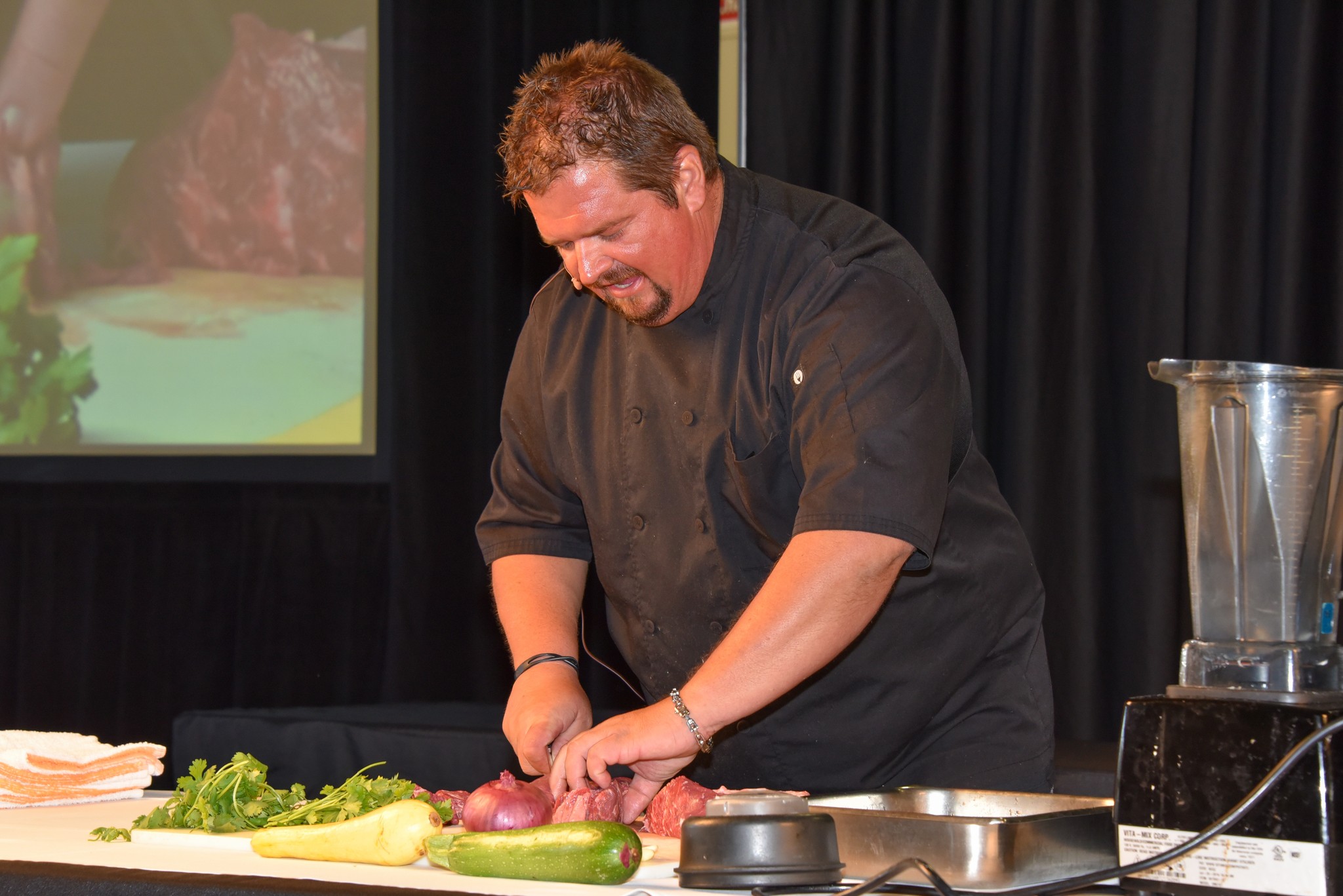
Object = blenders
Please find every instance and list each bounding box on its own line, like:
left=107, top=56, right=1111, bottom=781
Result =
left=1116, top=359, right=1343, bottom=896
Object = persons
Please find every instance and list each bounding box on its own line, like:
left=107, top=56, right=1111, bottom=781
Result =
left=0, top=0, right=369, bottom=297
left=475, top=39, right=1055, bottom=825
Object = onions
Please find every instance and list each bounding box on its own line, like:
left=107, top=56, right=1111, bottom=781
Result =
left=462, top=770, right=552, bottom=832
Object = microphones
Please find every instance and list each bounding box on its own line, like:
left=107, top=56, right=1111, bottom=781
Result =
left=563, top=260, right=582, bottom=290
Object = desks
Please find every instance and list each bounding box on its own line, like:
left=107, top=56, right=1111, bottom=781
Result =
left=171, top=702, right=631, bottom=792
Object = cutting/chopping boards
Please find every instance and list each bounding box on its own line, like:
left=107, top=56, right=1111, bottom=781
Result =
left=131, top=825, right=681, bottom=879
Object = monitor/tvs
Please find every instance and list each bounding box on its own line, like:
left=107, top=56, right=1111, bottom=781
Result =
left=0, top=0, right=391, bottom=484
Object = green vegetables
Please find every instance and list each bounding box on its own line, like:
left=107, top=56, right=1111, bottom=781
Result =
left=0, top=233, right=98, bottom=444
left=89, top=752, right=454, bottom=843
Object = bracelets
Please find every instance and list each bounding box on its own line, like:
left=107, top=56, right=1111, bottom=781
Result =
left=670, top=688, right=714, bottom=754
left=0, top=101, right=64, bottom=152
left=514, top=653, right=579, bottom=681
